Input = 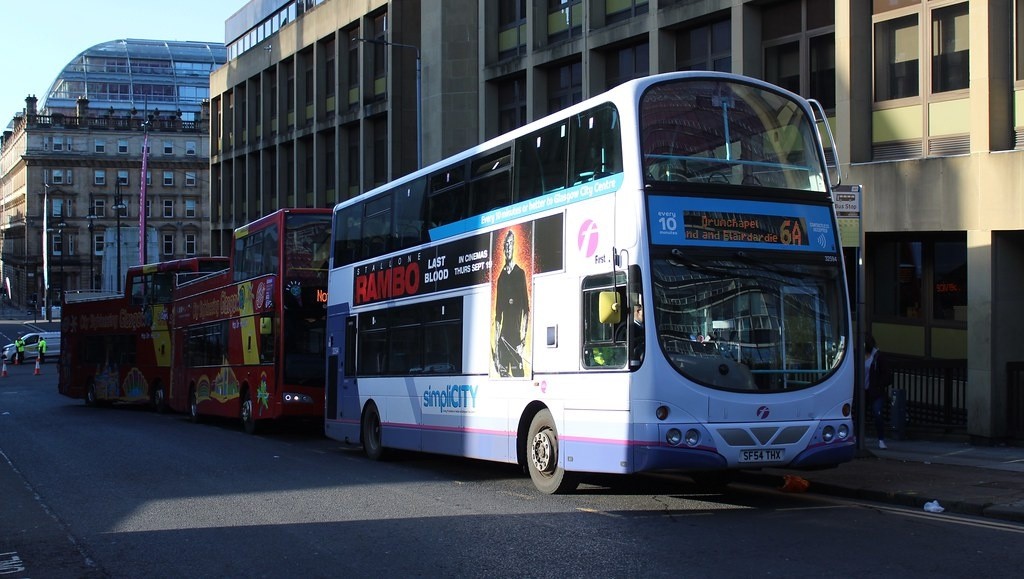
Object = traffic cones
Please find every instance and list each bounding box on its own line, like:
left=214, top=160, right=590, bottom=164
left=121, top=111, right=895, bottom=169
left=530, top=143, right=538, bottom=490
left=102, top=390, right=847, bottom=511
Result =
left=0, top=360, right=10, bottom=378
left=13, top=352, right=20, bottom=366
left=32, top=357, right=41, bottom=375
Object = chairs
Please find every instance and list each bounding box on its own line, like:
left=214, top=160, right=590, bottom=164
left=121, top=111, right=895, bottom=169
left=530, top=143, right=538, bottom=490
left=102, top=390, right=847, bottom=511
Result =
left=346, top=163, right=764, bottom=266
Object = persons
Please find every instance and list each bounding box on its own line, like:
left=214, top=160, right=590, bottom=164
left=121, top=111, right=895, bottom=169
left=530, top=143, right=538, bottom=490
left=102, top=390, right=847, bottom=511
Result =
left=38, top=337, right=47, bottom=364
left=866, top=334, right=895, bottom=450
left=15, top=336, right=26, bottom=364
left=492, top=231, right=530, bottom=378
left=615, top=304, right=645, bottom=358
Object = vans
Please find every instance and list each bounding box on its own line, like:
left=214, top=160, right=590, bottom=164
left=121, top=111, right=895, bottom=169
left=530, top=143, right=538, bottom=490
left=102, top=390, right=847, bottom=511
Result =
left=2, top=331, right=61, bottom=364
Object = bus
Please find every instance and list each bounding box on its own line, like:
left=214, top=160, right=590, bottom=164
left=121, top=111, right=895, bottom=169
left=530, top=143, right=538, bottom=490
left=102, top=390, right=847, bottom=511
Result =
left=324, top=70, right=858, bottom=497
left=58, top=255, right=230, bottom=414
left=168, top=205, right=333, bottom=435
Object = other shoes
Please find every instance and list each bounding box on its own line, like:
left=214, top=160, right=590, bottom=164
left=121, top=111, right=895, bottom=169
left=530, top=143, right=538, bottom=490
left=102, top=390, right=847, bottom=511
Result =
left=878, top=440, right=888, bottom=449
